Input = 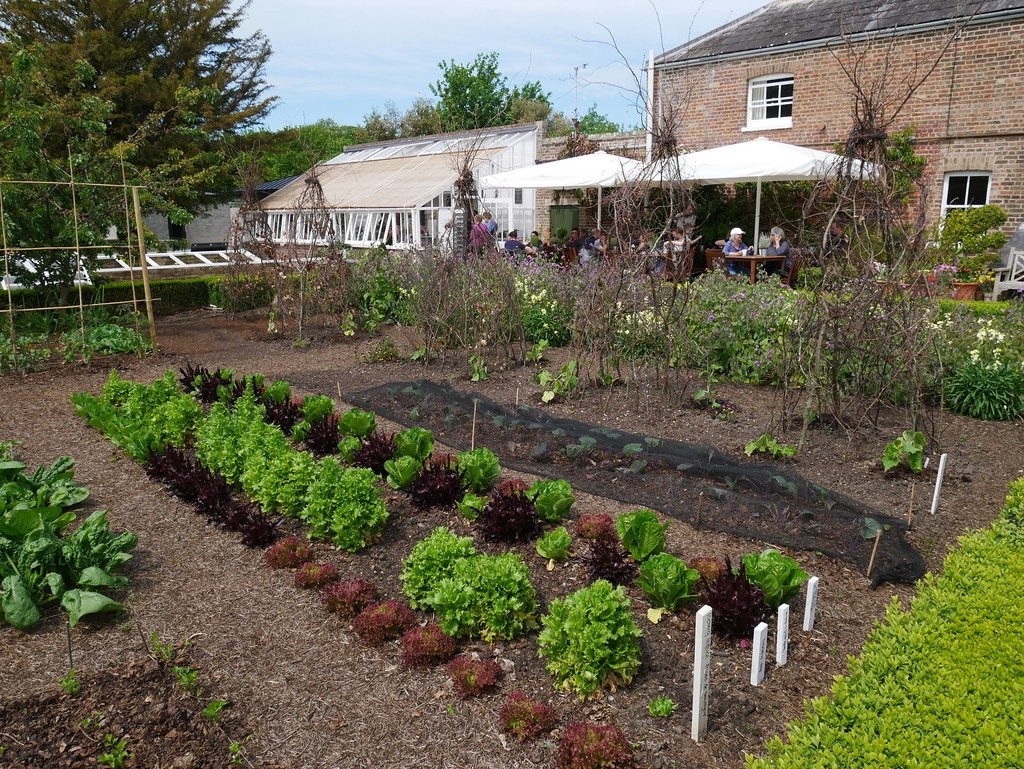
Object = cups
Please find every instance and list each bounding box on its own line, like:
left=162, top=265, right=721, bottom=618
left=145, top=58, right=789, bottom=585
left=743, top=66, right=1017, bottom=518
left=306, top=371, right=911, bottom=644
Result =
left=741, top=250, right=747, bottom=256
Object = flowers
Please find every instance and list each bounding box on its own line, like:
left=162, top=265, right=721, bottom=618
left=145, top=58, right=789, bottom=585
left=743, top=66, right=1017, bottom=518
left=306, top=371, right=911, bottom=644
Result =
left=910, top=257, right=998, bottom=284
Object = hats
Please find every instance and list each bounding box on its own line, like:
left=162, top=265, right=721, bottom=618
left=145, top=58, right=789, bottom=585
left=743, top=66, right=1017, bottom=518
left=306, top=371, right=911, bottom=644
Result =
left=730, top=228, right=745, bottom=234
left=531, top=231, right=538, bottom=236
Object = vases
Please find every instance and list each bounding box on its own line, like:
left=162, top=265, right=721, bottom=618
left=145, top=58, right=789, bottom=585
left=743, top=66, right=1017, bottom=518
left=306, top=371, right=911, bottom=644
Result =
left=953, top=282, right=984, bottom=301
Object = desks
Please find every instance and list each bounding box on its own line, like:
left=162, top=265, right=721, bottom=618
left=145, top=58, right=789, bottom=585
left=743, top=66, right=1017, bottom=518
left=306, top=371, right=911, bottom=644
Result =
left=725, top=254, right=786, bottom=286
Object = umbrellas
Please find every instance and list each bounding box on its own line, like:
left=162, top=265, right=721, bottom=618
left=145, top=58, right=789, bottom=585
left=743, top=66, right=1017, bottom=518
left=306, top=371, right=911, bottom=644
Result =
left=480, top=136, right=886, bottom=255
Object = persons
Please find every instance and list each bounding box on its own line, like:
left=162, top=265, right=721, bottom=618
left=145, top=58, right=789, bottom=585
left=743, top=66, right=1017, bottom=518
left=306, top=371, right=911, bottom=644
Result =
left=715, top=227, right=754, bottom=283
left=825, top=220, right=849, bottom=264
left=470, top=199, right=698, bottom=283
left=763, top=226, right=794, bottom=277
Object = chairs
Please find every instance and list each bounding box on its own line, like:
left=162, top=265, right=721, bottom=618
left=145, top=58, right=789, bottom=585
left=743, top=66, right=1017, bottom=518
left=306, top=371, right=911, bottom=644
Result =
left=502, top=243, right=805, bottom=291
left=992, top=246, right=1024, bottom=302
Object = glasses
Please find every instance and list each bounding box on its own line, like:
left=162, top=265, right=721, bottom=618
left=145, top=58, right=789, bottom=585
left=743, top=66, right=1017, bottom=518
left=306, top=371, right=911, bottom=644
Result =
left=572, top=232, right=578, bottom=234
